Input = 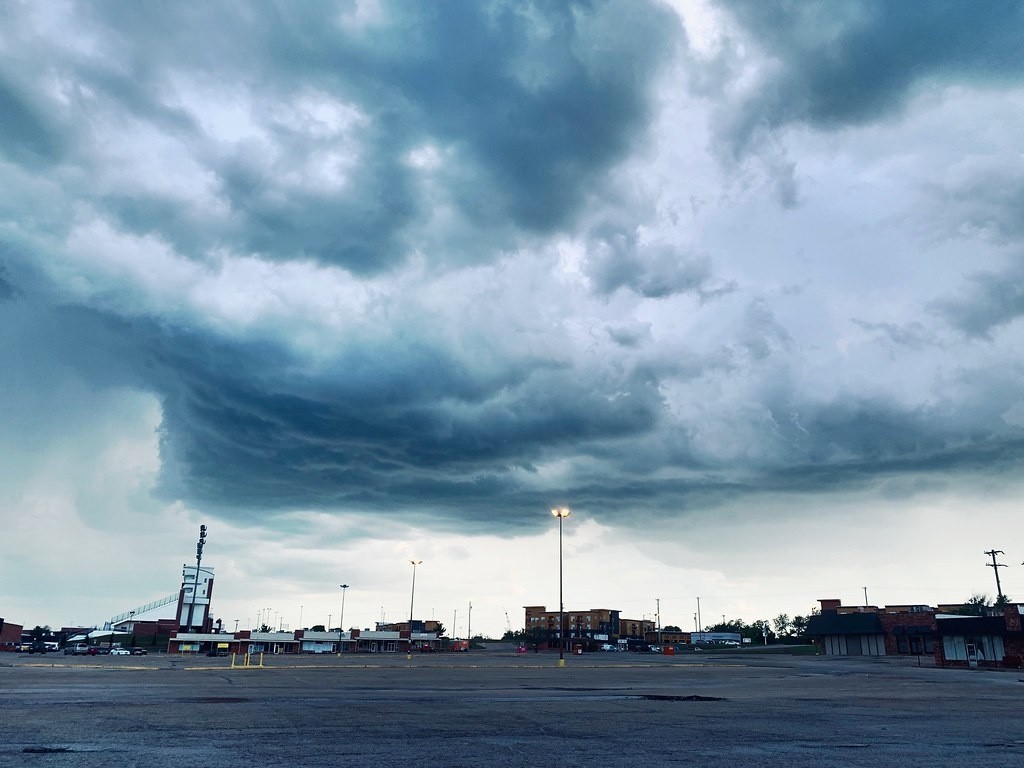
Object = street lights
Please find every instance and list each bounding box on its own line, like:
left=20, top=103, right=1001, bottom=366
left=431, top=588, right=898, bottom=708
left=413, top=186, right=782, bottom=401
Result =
left=338, top=584, right=350, bottom=659
left=406, top=560, right=422, bottom=662
left=551, top=508, right=571, bottom=669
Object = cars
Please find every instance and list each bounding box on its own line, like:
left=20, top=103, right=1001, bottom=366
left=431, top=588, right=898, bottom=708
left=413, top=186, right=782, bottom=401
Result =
left=88, top=646, right=109, bottom=656
left=132, top=646, right=148, bottom=656
left=601, top=645, right=618, bottom=652
left=63, top=643, right=89, bottom=656
left=2, top=640, right=60, bottom=654
left=110, top=648, right=131, bottom=656
